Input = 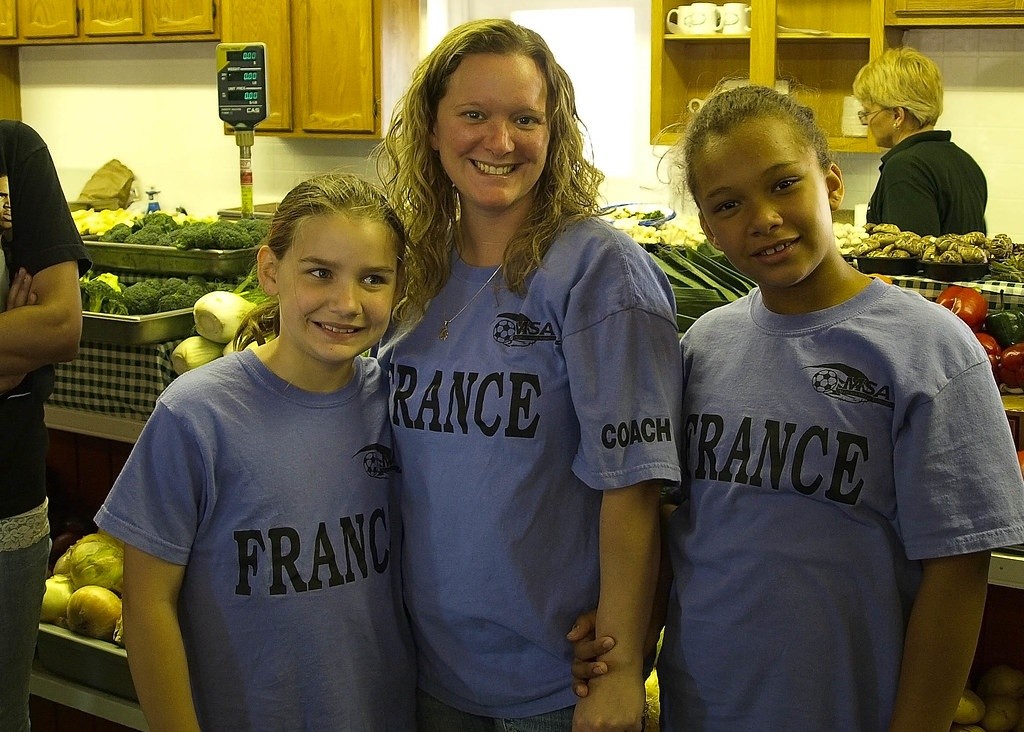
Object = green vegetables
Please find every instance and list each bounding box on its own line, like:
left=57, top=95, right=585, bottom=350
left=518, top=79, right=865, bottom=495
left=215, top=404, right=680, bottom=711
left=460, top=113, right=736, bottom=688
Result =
left=167, top=262, right=279, bottom=374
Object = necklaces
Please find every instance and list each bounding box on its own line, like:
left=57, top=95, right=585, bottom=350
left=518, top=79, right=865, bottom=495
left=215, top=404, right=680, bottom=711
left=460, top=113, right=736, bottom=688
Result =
left=439, top=263, right=503, bottom=341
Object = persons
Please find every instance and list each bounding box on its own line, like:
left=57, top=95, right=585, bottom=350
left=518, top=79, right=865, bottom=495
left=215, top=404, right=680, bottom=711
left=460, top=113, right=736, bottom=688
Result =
left=92, top=171, right=419, bottom=732
left=568, top=86, right=1024, bottom=731
left=375, top=19, right=684, bottom=732
left=0, top=119, right=92, bottom=732
left=852, top=47, right=987, bottom=239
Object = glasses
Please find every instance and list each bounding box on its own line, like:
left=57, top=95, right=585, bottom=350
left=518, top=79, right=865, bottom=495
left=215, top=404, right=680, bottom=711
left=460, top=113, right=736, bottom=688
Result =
left=858, top=106, right=908, bottom=125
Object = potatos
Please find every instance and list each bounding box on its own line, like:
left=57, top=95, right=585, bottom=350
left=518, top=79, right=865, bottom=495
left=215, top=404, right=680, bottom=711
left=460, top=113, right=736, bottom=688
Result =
left=947, top=664, right=1024, bottom=732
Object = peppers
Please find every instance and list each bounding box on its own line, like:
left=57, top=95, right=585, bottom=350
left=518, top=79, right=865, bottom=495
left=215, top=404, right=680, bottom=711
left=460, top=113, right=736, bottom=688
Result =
left=933, top=285, right=1024, bottom=390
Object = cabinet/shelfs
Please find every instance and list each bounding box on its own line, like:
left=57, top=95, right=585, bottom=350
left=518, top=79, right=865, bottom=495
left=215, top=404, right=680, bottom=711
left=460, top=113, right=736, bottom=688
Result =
left=650, top=0, right=1024, bottom=154
left=0, top=0, right=428, bottom=139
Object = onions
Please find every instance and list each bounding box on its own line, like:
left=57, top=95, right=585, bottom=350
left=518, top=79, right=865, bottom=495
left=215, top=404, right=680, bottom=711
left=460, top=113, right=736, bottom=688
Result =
left=32, top=526, right=126, bottom=646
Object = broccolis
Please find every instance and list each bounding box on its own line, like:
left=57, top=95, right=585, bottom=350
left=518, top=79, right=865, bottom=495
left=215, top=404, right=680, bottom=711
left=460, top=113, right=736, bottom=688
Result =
left=67, top=207, right=269, bottom=315
